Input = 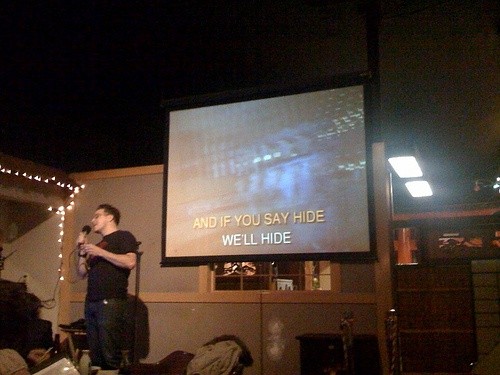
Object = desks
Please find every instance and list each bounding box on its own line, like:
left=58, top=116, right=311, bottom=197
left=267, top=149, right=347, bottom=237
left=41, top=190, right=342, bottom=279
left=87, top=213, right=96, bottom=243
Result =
left=295, top=332, right=379, bottom=375
left=62, top=329, right=87, bottom=364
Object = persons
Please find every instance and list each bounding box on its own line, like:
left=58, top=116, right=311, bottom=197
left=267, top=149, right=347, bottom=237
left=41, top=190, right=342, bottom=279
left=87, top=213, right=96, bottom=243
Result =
left=76, top=203, right=137, bottom=370
left=0, top=307, right=50, bottom=375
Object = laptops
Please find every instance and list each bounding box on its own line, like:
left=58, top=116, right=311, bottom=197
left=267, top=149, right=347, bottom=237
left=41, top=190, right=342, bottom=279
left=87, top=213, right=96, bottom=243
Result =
left=29, top=351, right=81, bottom=375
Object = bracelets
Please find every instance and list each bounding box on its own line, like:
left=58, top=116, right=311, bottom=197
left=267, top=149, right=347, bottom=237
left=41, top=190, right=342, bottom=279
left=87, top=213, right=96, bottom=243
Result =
left=78, top=252, right=86, bottom=258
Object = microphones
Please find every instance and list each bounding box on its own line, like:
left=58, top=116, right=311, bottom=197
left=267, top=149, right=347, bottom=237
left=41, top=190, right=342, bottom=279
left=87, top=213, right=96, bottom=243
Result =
left=77, top=225, right=91, bottom=249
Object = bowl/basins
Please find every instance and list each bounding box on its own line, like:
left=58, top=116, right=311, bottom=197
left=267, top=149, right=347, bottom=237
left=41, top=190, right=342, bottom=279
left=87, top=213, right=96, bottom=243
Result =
left=77, top=366, right=101, bottom=375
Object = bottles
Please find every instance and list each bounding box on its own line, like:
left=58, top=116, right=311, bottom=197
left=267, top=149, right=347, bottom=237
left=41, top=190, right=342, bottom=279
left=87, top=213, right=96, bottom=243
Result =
left=79, top=350, right=91, bottom=375
